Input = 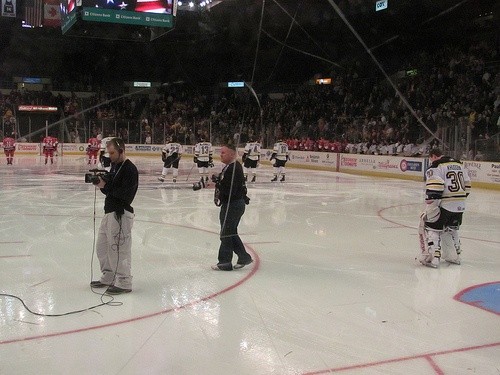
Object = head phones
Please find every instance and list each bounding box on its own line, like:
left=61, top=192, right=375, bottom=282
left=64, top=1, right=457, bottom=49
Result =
left=112, top=138, right=123, bottom=154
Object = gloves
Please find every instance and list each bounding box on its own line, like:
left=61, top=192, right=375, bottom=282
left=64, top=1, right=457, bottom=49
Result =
left=209, top=157, right=214, bottom=163
left=194, top=157, right=198, bottom=163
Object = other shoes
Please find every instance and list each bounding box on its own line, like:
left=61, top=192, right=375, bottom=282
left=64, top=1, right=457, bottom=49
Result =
left=234, top=258, right=253, bottom=269
left=212, top=264, right=219, bottom=270
left=106, top=286, right=131, bottom=294
left=90, top=281, right=108, bottom=288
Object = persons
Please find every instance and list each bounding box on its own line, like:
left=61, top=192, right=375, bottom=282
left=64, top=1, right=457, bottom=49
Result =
left=193, top=136, right=213, bottom=184
left=206, top=145, right=254, bottom=271
left=241, top=138, right=261, bottom=183
left=417, top=149, right=471, bottom=268
left=158, top=136, right=181, bottom=182
left=31, top=41, right=500, bottom=161
left=42, top=135, right=57, bottom=165
left=87, top=134, right=100, bottom=164
left=3, top=134, right=16, bottom=165
left=99, top=134, right=117, bottom=172
left=89, top=138, right=139, bottom=296
left=270, top=139, right=289, bottom=182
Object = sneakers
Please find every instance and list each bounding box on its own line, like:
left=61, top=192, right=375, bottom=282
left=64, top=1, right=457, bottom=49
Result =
left=251, top=178, right=255, bottom=184
left=158, top=177, right=164, bottom=182
left=280, top=178, right=285, bottom=182
left=244, top=177, right=247, bottom=183
left=271, top=177, right=277, bottom=182
left=173, top=178, right=176, bottom=182
